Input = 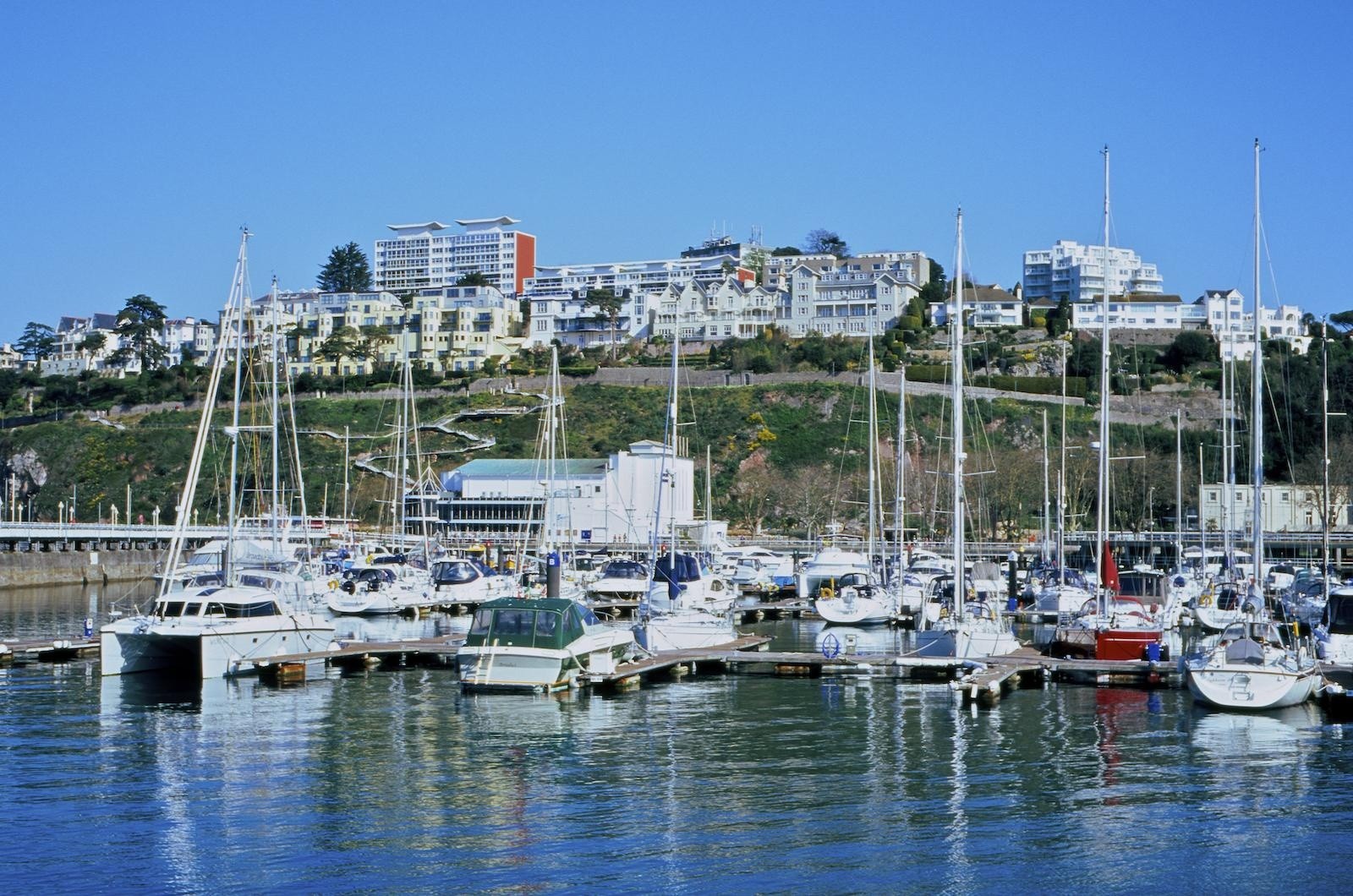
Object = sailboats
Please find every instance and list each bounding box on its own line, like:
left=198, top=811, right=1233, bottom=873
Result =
left=93, top=222, right=1353, bottom=719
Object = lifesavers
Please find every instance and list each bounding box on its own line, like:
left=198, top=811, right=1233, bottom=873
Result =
left=753, top=582, right=763, bottom=590
left=769, top=585, right=779, bottom=591
left=821, top=587, right=832, bottom=598
left=1199, top=595, right=1214, bottom=605
left=329, top=580, right=338, bottom=590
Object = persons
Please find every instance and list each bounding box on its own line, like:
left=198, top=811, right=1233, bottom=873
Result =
left=613, top=533, right=627, bottom=542
left=994, top=520, right=1019, bottom=544
